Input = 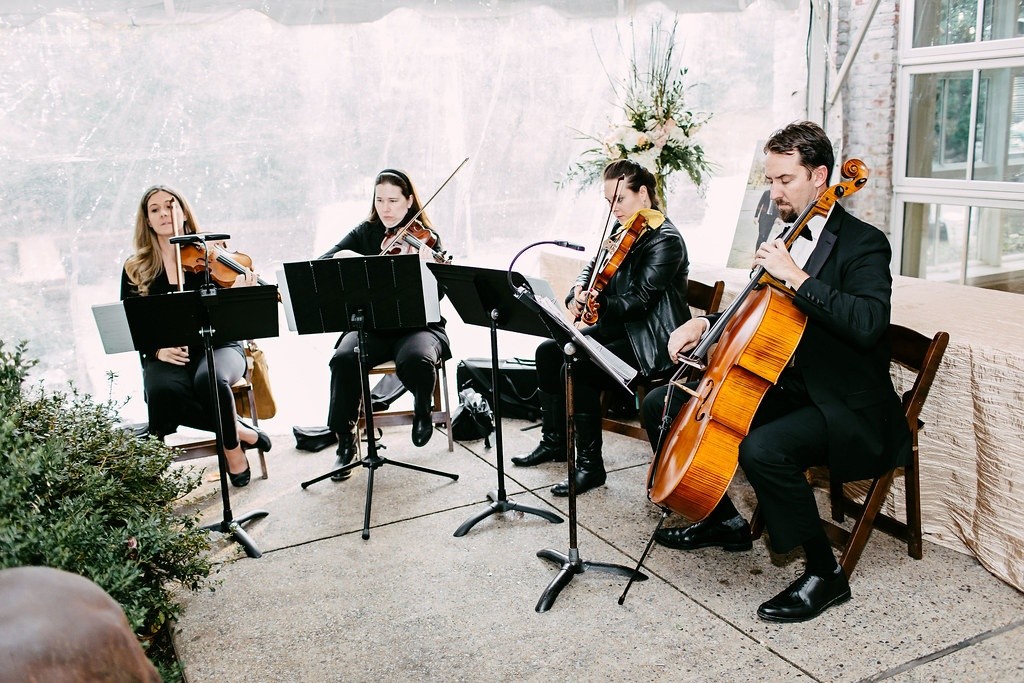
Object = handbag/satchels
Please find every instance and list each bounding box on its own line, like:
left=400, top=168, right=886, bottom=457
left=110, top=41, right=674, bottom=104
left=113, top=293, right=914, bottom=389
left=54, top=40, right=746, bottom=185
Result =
left=450, top=394, right=496, bottom=441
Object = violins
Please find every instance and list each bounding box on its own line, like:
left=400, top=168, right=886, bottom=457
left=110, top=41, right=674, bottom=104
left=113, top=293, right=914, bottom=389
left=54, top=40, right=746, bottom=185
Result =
left=581, top=213, right=645, bottom=326
left=180, top=232, right=282, bottom=302
left=380, top=219, right=453, bottom=264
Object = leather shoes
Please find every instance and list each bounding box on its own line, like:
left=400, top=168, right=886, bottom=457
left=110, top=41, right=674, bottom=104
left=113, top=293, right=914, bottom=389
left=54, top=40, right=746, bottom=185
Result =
left=331, top=447, right=358, bottom=481
left=653, top=518, right=753, bottom=552
left=511, top=441, right=568, bottom=467
left=225, top=451, right=252, bottom=488
left=410, top=399, right=433, bottom=447
left=550, top=466, right=607, bottom=497
left=757, top=563, right=851, bottom=622
left=236, top=414, right=272, bottom=452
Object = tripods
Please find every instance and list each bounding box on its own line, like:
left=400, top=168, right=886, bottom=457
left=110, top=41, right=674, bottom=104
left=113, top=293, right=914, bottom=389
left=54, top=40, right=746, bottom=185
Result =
left=123, top=284, right=280, bottom=559
left=284, top=254, right=458, bottom=540
left=427, top=263, right=564, bottom=537
left=535, top=307, right=649, bottom=613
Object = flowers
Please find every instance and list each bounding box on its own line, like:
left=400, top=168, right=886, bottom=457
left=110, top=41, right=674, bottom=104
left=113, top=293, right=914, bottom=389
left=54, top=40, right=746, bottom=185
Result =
left=554, top=10, right=713, bottom=220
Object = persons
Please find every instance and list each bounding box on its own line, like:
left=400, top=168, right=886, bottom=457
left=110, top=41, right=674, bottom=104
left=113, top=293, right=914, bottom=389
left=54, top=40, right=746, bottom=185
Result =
left=510, top=161, right=691, bottom=496
left=121, top=185, right=272, bottom=486
left=318, top=168, right=452, bottom=481
left=640, top=120, right=913, bottom=622
left=754, top=190, right=777, bottom=253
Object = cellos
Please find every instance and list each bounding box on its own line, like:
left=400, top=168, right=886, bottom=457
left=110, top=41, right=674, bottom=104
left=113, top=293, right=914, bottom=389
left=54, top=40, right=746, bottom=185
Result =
left=618, top=158, right=868, bottom=605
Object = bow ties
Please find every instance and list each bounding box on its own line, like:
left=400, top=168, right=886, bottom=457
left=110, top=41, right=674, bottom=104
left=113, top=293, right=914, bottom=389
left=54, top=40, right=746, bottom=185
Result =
left=783, top=224, right=813, bottom=242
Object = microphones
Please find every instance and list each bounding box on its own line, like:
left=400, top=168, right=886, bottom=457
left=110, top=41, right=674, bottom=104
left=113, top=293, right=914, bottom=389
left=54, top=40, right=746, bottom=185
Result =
left=507, top=240, right=585, bottom=292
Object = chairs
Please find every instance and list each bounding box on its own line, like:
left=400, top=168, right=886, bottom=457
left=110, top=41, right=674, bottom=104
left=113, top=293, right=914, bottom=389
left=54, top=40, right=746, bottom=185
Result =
left=601, top=280, right=725, bottom=444
left=353, top=357, right=454, bottom=453
left=750, top=321, right=950, bottom=578
left=140, top=336, right=268, bottom=481
